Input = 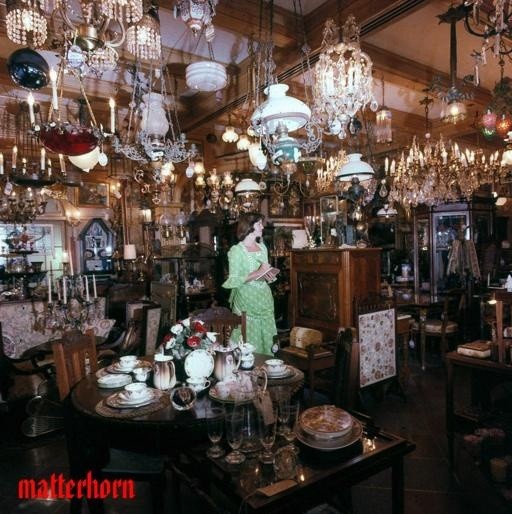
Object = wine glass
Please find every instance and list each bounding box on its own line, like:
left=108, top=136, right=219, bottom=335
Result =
left=261, top=391, right=298, bottom=464
left=205, top=406, right=246, bottom=466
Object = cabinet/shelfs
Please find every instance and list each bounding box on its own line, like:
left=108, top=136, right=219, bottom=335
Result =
left=287, top=246, right=383, bottom=402
left=412, top=202, right=472, bottom=304
left=444, top=339, right=512, bottom=474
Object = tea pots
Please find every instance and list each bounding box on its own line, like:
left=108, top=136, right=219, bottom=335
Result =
left=230, top=367, right=269, bottom=453
left=152, top=354, right=178, bottom=389
left=213, top=345, right=241, bottom=380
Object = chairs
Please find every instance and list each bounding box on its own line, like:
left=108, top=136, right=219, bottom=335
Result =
left=51, top=328, right=98, bottom=402
left=305, top=325, right=358, bottom=411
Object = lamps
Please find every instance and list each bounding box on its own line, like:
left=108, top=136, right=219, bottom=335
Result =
left=0, top=1, right=512, bottom=239
left=123, top=243, right=136, bottom=282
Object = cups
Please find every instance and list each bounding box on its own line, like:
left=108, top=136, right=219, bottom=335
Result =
left=124, top=382, right=151, bottom=399
left=401, top=263, right=411, bottom=278
left=131, top=367, right=152, bottom=381
left=185, top=377, right=210, bottom=392
left=241, top=353, right=255, bottom=370
left=120, top=356, right=139, bottom=367
left=274, top=449, right=298, bottom=480
left=170, top=388, right=199, bottom=411
left=264, top=358, right=287, bottom=373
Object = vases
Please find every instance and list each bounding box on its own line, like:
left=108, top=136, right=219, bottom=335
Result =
left=172, top=359, right=189, bottom=383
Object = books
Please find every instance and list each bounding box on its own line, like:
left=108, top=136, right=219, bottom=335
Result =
left=256, top=267, right=281, bottom=281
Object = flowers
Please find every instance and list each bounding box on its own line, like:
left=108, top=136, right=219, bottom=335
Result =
left=161, top=314, right=220, bottom=361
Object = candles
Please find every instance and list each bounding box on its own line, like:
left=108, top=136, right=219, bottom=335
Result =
left=48, top=273, right=98, bottom=304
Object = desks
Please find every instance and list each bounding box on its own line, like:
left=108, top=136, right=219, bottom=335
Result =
left=277, top=345, right=335, bottom=392
left=183, top=394, right=417, bottom=512
left=382, top=293, right=453, bottom=370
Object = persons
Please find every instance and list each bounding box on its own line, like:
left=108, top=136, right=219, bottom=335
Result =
left=221, top=212, right=278, bottom=358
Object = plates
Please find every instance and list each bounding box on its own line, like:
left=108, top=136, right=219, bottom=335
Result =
left=103, top=386, right=163, bottom=408
left=267, top=366, right=294, bottom=379
left=297, top=416, right=364, bottom=451
left=184, top=348, right=214, bottom=378
left=104, top=360, right=152, bottom=374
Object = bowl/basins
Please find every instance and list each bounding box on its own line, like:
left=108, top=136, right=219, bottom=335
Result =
left=300, top=404, right=353, bottom=438
